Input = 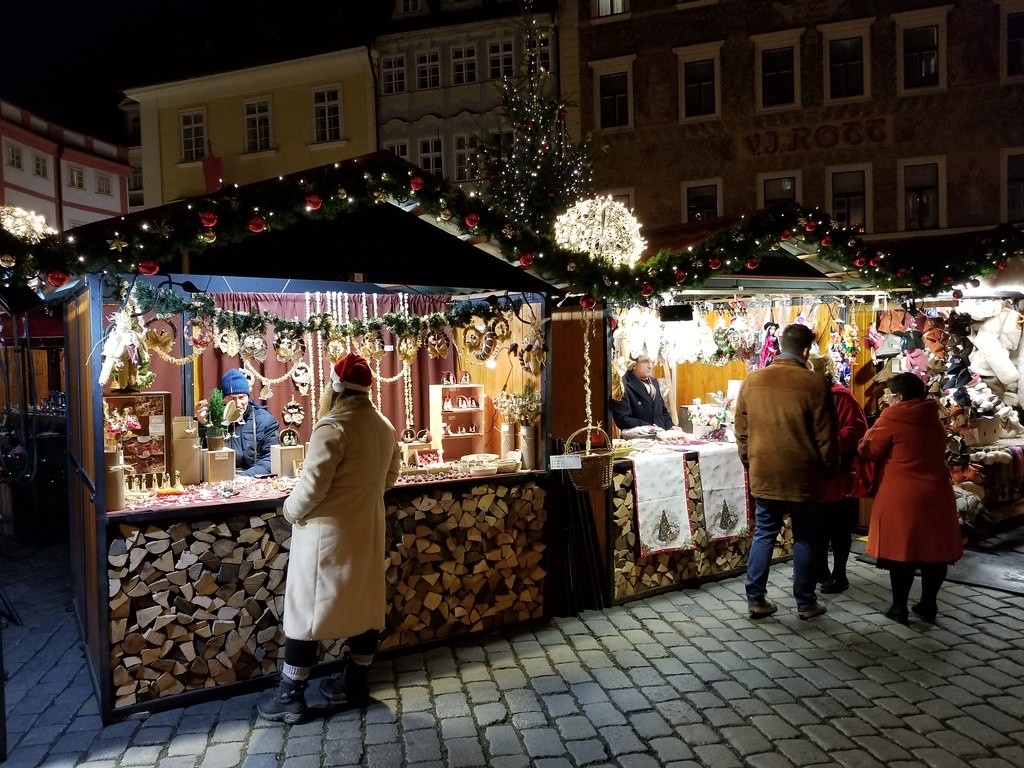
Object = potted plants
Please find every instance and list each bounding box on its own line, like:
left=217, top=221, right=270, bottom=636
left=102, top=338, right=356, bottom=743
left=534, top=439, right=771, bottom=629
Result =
left=205, top=388, right=227, bottom=451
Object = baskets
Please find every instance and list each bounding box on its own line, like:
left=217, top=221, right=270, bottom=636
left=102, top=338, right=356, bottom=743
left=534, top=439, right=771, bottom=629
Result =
left=562, top=426, right=614, bottom=491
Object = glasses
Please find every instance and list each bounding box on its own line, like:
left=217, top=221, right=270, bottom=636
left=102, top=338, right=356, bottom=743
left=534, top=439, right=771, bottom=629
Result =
left=884, top=392, right=898, bottom=402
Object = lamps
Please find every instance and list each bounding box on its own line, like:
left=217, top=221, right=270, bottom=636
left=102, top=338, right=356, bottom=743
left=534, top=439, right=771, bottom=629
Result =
left=658, top=304, right=694, bottom=322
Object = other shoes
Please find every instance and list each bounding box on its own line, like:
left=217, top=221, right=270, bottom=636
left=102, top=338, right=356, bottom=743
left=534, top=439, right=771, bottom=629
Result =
left=883, top=605, right=909, bottom=624
left=912, top=602, right=938, bottom=622
left=867, top=309, right=1005, bottom=433
left=747, top=601, right=776, bottom=619
left=833, top=576, right=850, bottom=592
left=820, top=575, right=834, bottom=594
left=799, top=599, right=826, bottom=619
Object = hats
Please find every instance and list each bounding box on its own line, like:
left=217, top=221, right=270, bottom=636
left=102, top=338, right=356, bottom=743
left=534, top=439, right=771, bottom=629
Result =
left=220, top=369, right=249, bottom=399
left=330, top=351, right=373, bottom=393
left=627, top=342, right=650, bottom=370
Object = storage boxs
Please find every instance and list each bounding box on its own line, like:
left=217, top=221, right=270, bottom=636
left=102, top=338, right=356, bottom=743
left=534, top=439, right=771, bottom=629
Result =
left=270, top=444, right=305, bottom=480
left=962, top=415, right=1000, bottom=446
left=173, top=420, right=200, bottom=439
left=172, top=437, right=202, bottom=485
left=677, top=404, right=717, bottom=434
left=200, top=447, right=236, bottom=486
left=413, top=449, right=440, bottom=466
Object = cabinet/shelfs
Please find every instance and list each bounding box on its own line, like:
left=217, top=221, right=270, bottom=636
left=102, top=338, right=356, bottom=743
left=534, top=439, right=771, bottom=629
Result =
left=429, top=383, right=485, bottom=463
left=103, top=390, right=173, bottom=488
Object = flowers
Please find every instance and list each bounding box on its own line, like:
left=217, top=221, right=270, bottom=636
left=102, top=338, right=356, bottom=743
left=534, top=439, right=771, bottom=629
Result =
left=514, top=379, right=543, bottom=427
left=490, top=389, right=517, bottom=422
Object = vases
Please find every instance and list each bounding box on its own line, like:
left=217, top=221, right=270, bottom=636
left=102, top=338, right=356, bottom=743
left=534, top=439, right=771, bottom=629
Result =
left=519, top=427, right=536, bottom=469
left=501, top=423, right=514, bottom=459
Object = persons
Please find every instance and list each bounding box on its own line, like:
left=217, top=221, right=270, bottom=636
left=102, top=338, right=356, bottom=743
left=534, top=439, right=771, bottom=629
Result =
left=194, top=368, right=279, bottom=479
left=803, top=354, right=875, bottom=592
left=858, top=372, right=965, bottom=619
left=733, top=323, right=843, bottom=620
left=255, top=353, right=400, bottom=723
left=613, top=350, right=683, bottom=440
left=760, top=322, right=781, bottom=366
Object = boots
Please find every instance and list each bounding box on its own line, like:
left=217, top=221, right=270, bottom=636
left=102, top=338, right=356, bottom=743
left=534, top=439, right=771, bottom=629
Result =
left=320, top=659, right=375, bottom=700
left=257, top=673, right=310, bottom=724
